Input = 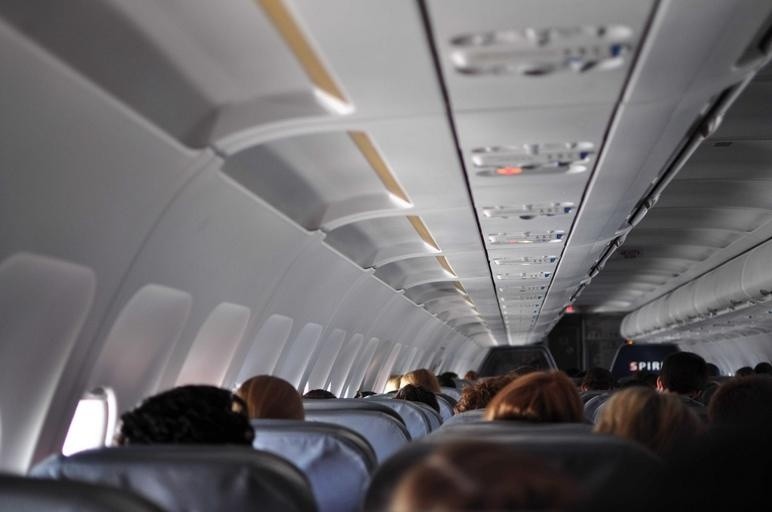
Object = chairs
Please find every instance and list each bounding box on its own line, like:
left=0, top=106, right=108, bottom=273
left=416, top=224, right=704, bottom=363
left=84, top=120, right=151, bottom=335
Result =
left=29, top=377, right=676, bottom=512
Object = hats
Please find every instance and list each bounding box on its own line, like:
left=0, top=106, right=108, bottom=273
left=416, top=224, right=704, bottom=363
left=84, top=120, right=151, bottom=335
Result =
left=115, top=385, right=254, bottom=445
